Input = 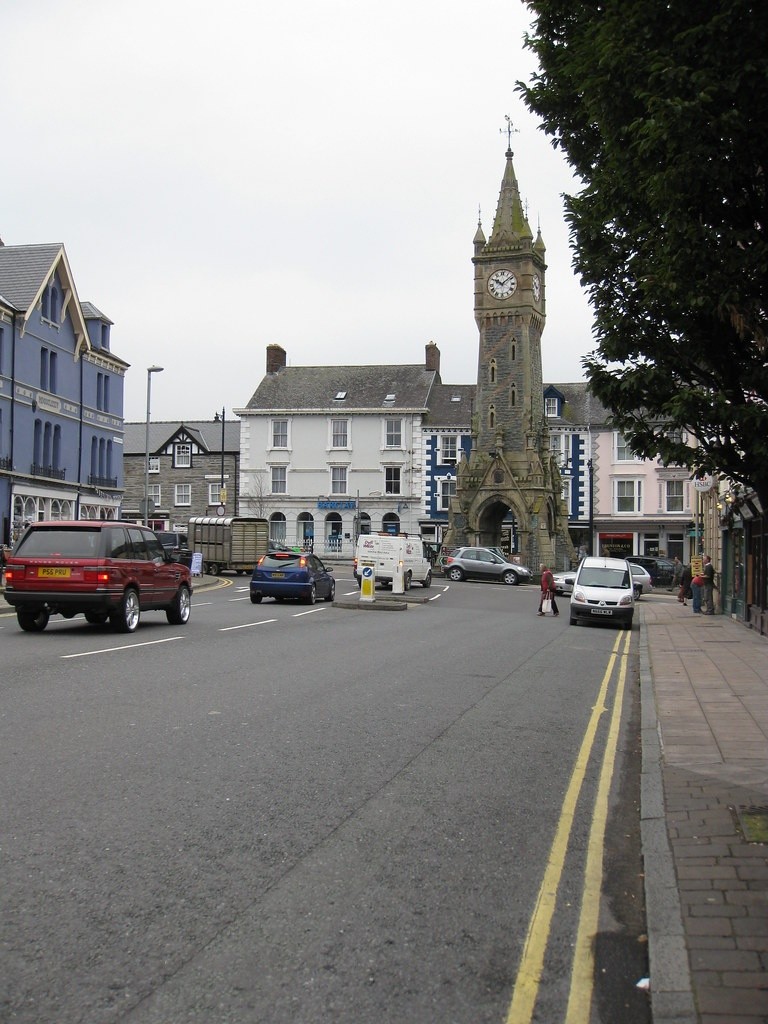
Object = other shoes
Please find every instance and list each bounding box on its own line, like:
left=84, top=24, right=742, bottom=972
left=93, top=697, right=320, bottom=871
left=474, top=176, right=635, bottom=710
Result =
left=666, top=588, right=673, bottom=592
left=704, top=608, right=714, bottom=615
left=694, top=609, right=704, bottom=615
left=552, top=612, right=559, bottom=617
left=537, top=612, right=545, bottom=616
left=683, top=603, right=687, bottom=606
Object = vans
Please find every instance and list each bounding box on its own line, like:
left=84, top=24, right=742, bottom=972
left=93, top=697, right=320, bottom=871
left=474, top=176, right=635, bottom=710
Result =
left=564, top=556, right=635, bottom=630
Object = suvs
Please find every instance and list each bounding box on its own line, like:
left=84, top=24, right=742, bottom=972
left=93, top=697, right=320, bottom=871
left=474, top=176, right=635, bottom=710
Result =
left=3, top=520, right=194, bottom=634
left=150, top=531, right=188, bottom=567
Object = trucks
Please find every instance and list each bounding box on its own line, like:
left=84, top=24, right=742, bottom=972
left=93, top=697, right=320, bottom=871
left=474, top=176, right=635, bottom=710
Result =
left=186, top=516, right=269, bottom=576
left=353, top=533, right=433, bottom=591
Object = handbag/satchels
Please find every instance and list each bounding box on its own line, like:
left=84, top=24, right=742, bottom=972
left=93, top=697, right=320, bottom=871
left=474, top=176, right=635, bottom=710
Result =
left=542, top=591, right=551, bottom=613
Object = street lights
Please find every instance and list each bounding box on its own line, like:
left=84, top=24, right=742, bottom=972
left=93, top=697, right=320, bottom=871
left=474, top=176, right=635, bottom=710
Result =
left=143, top=366, right=163, bottom=532
left=212, top=406, right=226, bottom=518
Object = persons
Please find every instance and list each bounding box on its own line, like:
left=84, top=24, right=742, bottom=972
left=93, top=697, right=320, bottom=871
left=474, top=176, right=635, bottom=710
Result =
left=696, top=556, right=714, bottom=615
left=666, top=556, right=683, bottom=592
left=536, top=563, right=560, bottom=616
left=680, top=565, right=719, bottom=612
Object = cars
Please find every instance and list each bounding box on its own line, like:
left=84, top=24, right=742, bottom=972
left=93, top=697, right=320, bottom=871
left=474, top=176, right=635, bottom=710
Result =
left=249, top=551, right=335, bottom=605
left=624, top=556, right=682, bottom=587
left=267, top=540, right=294, bottom=553
left=550, top=562, right=652, bottom=601
left=439, top=546, right=534, bottom=586
